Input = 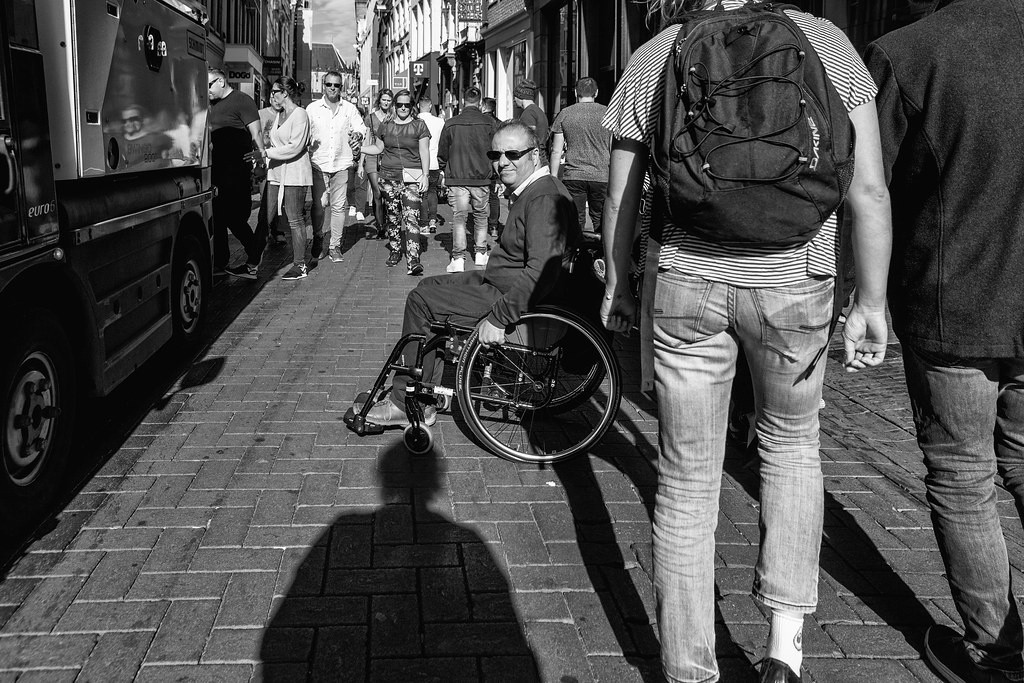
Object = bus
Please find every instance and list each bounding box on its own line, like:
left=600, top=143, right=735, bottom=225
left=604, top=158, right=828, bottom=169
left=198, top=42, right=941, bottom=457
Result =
left=0, top=0, right=263, bottom=500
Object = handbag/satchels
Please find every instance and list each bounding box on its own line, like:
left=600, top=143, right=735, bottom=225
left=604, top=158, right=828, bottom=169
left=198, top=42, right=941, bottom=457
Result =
left=377, top=153, right=384, bottom=172
left=402, top=168, right=423, bottom=182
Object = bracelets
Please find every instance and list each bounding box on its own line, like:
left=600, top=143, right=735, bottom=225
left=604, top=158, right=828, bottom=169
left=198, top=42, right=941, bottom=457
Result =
left=424, top=173, right=430, bottom=178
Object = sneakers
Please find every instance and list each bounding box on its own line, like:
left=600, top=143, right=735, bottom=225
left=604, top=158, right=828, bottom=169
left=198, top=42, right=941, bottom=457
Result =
left=311, top=238, right=324, bottom=259
left=924, top=622, right=993, bottom=683
left=281, top=264, right=307, bottom=280
left=255, top=250, right=265, bottom=267
left=475, top=252, right=490, bottom=266
left=446, top=258, right=464, bottom=272
left=328, top=246, right=343, bottom=262
left=385, top=249, right=402, bottom=267
left=225, top=263, right=258, bottom=279
left=352, top=388, right=437, bottom=427
left=213, top=264, right=229, bottom=275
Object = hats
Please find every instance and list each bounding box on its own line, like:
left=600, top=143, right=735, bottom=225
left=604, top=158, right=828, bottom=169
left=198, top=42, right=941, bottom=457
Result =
left=512, top=79, right=537, bottom=101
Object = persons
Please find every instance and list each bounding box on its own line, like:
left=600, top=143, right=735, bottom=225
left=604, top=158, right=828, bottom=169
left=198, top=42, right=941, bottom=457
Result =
left=437, top=86, right=498, bottom=273
left=209, top=67, right=366, bottom=279
left=514, top=80, right=549, bottom=166
left=347, top=87, right=444, bottom=275
left=481, top=97, right=500, bottom=236
left=352, top=117, right=581, bottom=426
left=600, top=0, right=1024, bottom=682
left=114, top=104, right=173, bottom=168
left=549, top=77, right=611, bottom=268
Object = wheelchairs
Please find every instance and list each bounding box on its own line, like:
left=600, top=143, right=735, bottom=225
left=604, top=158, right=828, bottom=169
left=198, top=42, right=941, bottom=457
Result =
left=342, top=228, right=621, bottom=463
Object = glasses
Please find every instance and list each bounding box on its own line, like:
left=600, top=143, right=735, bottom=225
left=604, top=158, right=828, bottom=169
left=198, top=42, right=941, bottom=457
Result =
left=324, top=82, right=341, bottom=88
left=395, top=102, right=412, bottom=108
left=486, top=147, right=537, bottom=161
left=271, top=89, right=282, bottom=95
left=209, top=78, right=219, bottom=89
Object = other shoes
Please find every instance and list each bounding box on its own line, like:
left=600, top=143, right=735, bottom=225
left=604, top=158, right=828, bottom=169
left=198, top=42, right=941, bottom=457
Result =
left=271, top=230, right=286, bottom=241
left=348, top=206, right=497, bottom=237
left=757, top=658, right=803, bottom=683
left=407, top=263, right=424, bottom=274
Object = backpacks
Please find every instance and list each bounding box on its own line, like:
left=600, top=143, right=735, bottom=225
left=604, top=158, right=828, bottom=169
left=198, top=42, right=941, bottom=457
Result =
left=647, top=0, right=855, bottom=241
